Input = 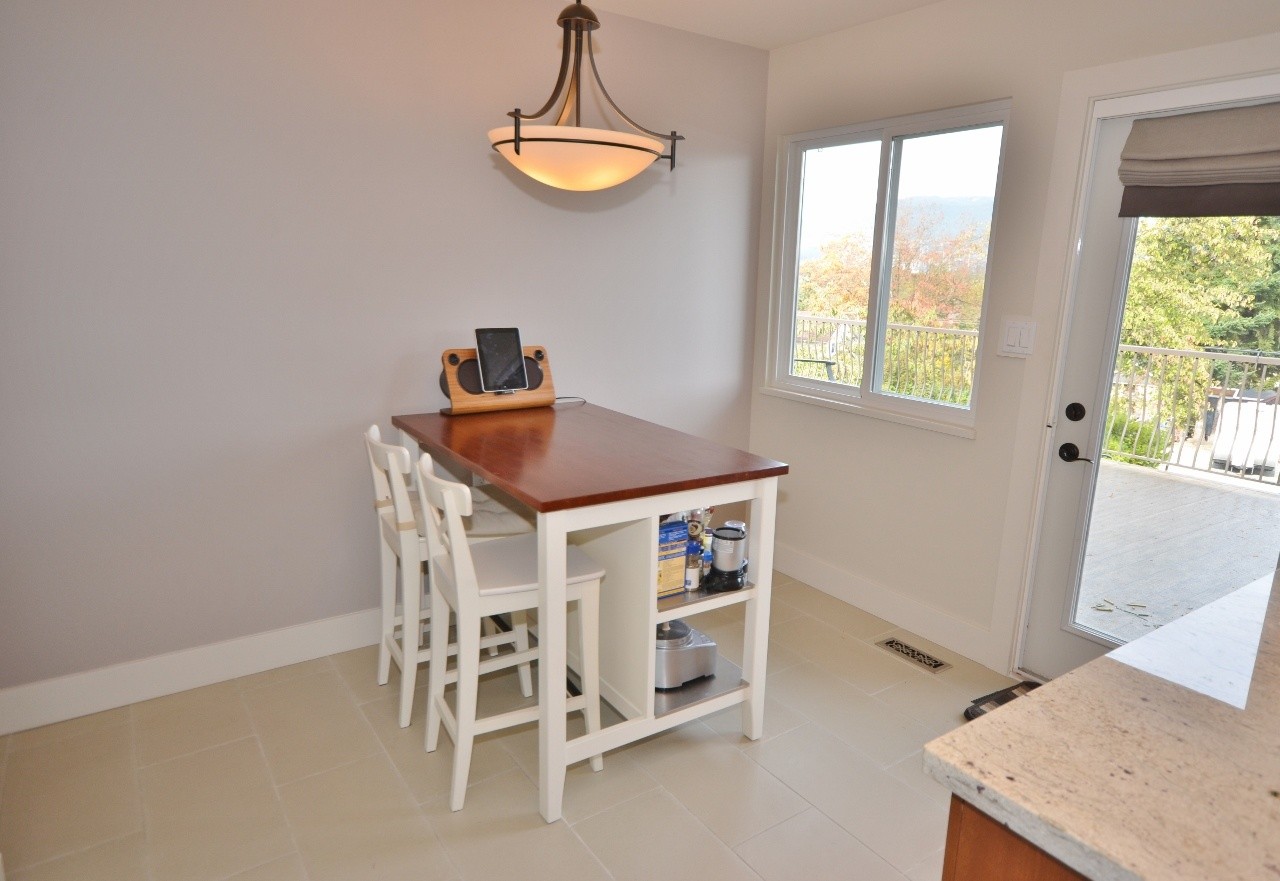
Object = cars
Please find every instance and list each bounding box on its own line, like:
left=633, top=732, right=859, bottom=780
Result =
left=1212, top=401, right=1279, bottom=472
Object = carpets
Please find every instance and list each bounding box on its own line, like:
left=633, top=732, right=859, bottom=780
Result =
left=962, top=681, right=1043, bottom=721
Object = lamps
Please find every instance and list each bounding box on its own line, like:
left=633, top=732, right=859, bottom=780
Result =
left=486, top=0, right=686, bottom=193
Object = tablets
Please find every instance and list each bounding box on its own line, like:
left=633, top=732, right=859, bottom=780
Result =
left=474, top=327, right=529, bottom=392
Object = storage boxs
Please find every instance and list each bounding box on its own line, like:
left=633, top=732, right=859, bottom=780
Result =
left=658, top=520, right=690, bottom=600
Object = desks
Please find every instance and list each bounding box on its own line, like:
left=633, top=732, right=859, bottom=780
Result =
left=391, top=400, right=788, bottom=825
left=921, top=570, right=1280, bottom=881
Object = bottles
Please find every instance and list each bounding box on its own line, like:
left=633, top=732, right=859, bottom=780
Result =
left=685, top=506, right=749, bottom=593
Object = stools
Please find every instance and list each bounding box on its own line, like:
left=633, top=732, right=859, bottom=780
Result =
left=411, top=453, right=607, bottom=814
left=363, top=424, right=534, bottom=728
